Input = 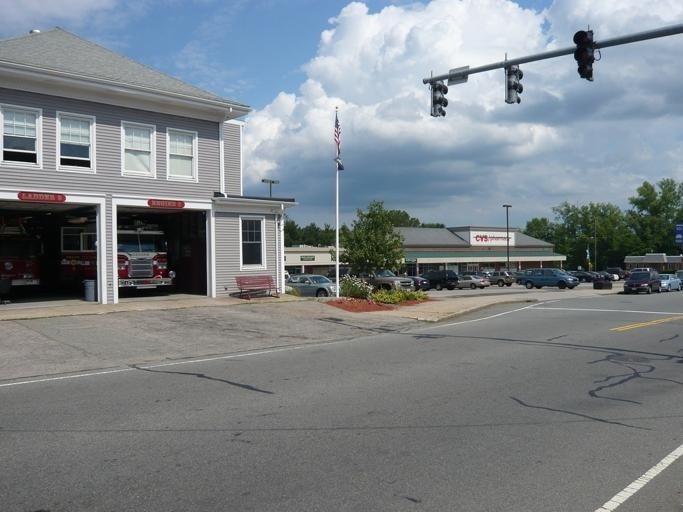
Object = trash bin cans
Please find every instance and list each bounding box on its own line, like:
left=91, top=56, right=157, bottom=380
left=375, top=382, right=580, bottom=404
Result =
left=82, top=280, right=97, bottom=301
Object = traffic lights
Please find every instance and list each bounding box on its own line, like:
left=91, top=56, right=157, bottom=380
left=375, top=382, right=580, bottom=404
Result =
left=506, top=67, right=524, bottom=104
left=432, top=81, right=449, bottom=117
left=572, top=29, right=595, bottom=81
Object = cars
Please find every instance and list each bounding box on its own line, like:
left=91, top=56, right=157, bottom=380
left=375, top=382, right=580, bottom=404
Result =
left=457, top=275, right=491, bottom=289
left=402, top=275, right=430, bottom=292
left=285, top=274, right=342, bottom=298
left=658, top=274, right=682, bottom=292
left=487, top=271, right=513, bottom=288
left=516, top=268, right=579, bottom=289
left=624, top=270, right=663, bottom=295
left=567, top=267, right=683, bottom=290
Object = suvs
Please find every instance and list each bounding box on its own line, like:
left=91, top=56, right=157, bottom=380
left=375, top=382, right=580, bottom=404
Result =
left=327, top=266, right=415, bottom=292
left=418, top=270, right=459, bottom=291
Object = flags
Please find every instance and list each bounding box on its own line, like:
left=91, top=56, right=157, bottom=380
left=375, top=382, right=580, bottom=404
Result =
left=335, top=112, right=343, bottom=170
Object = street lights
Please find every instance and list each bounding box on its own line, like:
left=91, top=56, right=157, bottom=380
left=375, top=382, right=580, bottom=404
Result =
left=262, top=179, right=280, bottom=199
left=502, top=204, right=512, bottom=271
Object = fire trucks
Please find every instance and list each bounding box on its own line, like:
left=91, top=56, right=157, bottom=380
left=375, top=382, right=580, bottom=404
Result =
left=0, top=216, right=176, bottom=300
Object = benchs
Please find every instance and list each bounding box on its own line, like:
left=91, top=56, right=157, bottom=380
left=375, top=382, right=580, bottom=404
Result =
left=234, top=275, right=280, bottom=301
